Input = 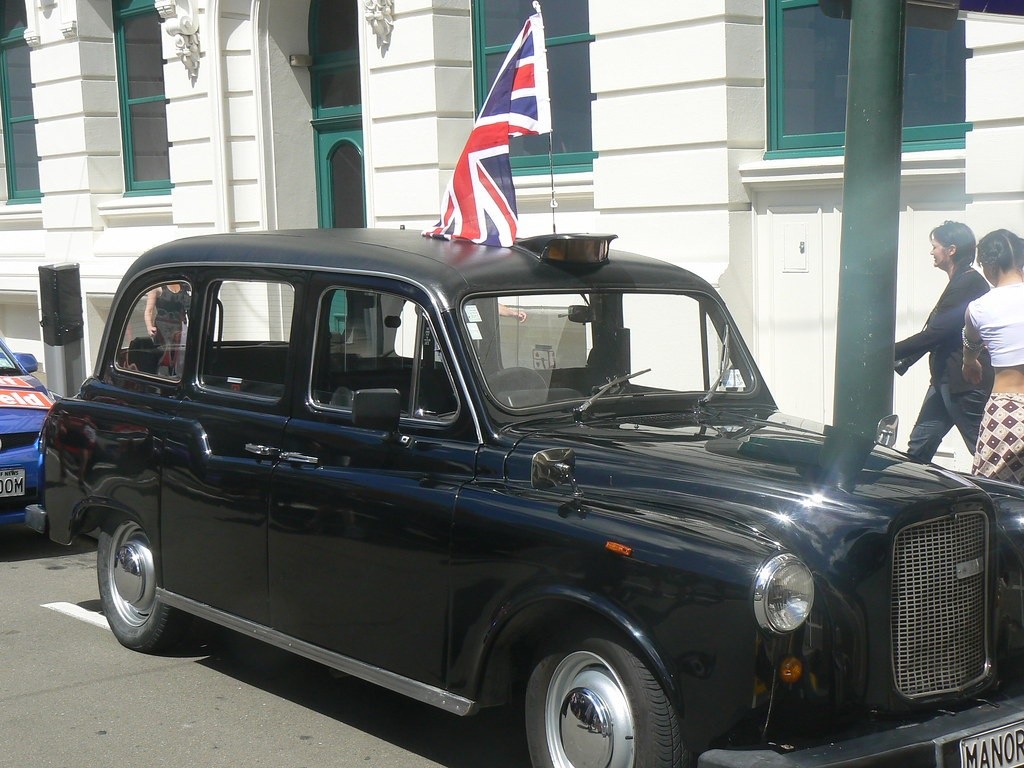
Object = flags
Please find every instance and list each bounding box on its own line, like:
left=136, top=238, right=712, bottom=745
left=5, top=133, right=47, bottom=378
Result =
left=421, top=12, right=552, bottom=249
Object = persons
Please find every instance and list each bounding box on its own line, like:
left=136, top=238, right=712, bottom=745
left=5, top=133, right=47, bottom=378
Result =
left=392, top=299, right=527, bottom=363
left=960, top=230, right=1024, bottom=485
left=144, top=283, right=191, bottom=376
left=895, top=222, right=995, bottom=465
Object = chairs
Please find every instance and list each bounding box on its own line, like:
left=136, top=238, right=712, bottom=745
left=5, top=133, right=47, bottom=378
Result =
left=126, top=335, right=158, bottom=372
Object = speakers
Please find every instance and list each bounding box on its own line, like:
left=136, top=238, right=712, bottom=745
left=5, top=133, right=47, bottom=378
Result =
left=37, top=263, right=84, bottom=347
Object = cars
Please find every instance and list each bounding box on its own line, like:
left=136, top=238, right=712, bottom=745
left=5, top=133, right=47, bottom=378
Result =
left=21, top=224, right=1024, bottom=768
left=0, top=335, right=59, bottom=526
